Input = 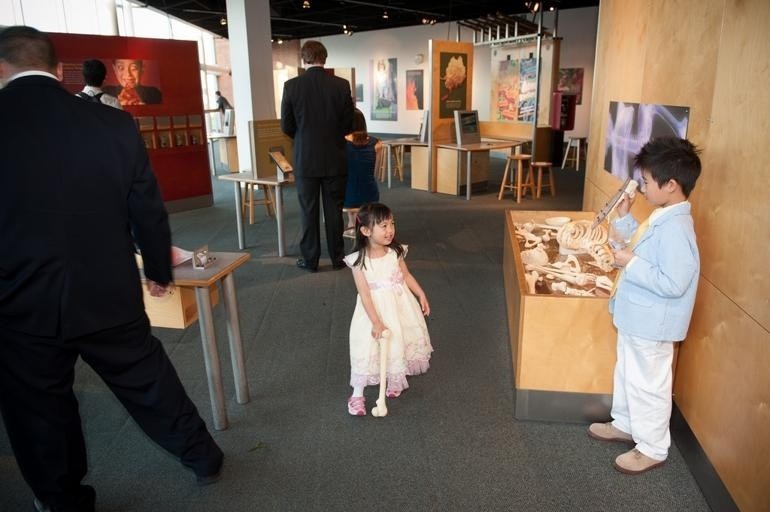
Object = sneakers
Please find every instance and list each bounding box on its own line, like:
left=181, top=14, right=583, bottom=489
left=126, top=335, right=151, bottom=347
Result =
left=385, top=386, right=401, bottom=397
left=614, top=448, right=668, bottom=474
left=348, top=396, right=366, bottom=415
left=588, top=420, right=637, bottom=449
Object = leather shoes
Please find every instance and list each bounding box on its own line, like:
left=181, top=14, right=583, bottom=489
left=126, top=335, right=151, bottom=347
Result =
left=333, top=256, right=347, bottom=269
left=33, top=496, right=45, bottom=512
left=194, top=474, right=221, bottom=483
left=297, top=257, right=317, bottom=273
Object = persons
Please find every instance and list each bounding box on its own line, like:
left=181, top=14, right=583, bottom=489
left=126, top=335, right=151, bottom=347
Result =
left=214, top=91, right=234, bottom=115
left=345, top=108, right=382, bottom=239
left=73, top=59, right=122, bottom=110
left=0, top=26, right=223, bottom=512
left=589, top=135, right=700, bottom=476
left=102, top=59, right=162, bottom=105
left=280, top=49, right=352, bottom=274
left=343, top=203, right=433, bottom=416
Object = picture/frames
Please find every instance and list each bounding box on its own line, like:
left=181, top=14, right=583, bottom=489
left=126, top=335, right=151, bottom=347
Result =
left=406, top=69, right=424, bottom=111
left=518, top=58, right=541, bottom=123
left=356, top=84, right=363, bottom=102
left=603, top=100, right=688, bottom=196
left=368, top=58, right=397, bottom=121
left=497, top=60, right=519, bottom=123
left=439, top=52, right=468, bottom=119
left=558, top=67, right=584, bottom=105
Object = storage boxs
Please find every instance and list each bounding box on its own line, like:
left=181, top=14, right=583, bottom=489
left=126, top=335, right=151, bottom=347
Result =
left=502, top=208, right=622, bottom=425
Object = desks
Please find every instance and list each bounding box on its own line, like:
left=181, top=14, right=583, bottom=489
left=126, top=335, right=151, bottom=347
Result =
left=217, top=172, right=296, bottom=258
left=137, top=248, right=251, bottom=432
left=208, top=135, right=237, bottom=177
left=382, top=136, right=429, bottom=189
left=435, top=135, right=532, bottom=201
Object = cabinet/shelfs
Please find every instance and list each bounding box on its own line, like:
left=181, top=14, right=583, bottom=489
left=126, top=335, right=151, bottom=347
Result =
left=552, top=90, right=575, bottom=131
left=436, top=147, right=490, bottom=197
left=410, top=146, right=432, bottom=192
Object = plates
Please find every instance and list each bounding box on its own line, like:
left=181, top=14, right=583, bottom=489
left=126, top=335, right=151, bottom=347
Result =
left=545, top=217, right=571, bottom=226
left=137, top=247, right=196, bottom=266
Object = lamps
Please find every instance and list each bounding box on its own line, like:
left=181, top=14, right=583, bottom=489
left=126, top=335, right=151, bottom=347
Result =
left=277, top=38, right=283, bottom=45
left=525, top=1, right=539, bottom=16
left=382, top=11, right=390, bottom=22
left=421, top=18, right=437, bottom=26
left=549, top=6, right=554, bottom=12
left=219, top=16, right=227, bottom=25
left=341, top=25, right=353, bottom=36
left=302, top=0, right=311, bottom=9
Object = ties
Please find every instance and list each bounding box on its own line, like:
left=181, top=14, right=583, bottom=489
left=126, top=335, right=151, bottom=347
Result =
left=610, top=216, right=648, bottom=299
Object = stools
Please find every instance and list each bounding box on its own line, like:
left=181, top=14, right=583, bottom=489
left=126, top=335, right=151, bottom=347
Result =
left=560, top=135, right=587, bottom=172
left=242, top=182, right=276, bottom=224
left=498, top=154, right=536, bottom=204
left=522, top=162, right=556, bottom=199
